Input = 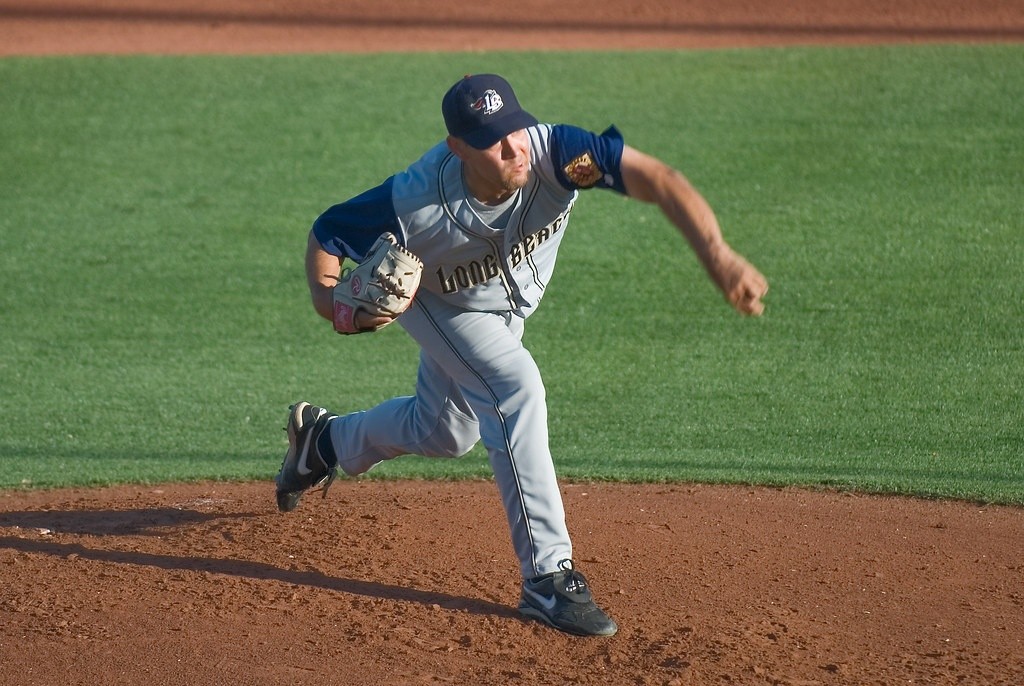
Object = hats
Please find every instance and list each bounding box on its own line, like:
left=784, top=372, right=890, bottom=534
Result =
left=442, top=74, right=539, bottom=150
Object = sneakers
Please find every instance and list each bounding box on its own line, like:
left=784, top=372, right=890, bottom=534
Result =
left=275, top=402, right=339, bottom=512
left=516, top=558, right=617, bottom=636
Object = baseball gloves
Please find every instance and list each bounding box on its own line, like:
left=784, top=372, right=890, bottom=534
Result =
left=323, top=231, right=424, bottom=336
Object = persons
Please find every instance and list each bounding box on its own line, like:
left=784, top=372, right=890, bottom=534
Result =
left=275, top=73, right=769, bottom=639
